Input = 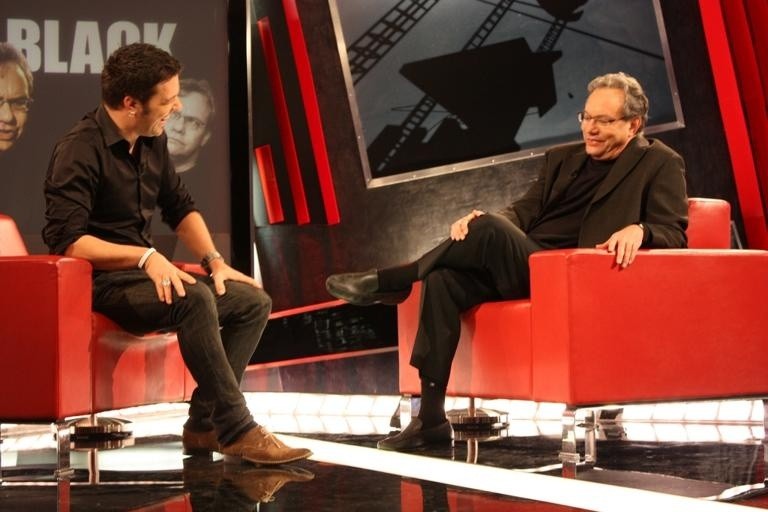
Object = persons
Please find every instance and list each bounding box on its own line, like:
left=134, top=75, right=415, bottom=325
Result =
left=2, top=41, right=33, bottom=216
left=38, top=42, right=314, bottom=463
left=324, top=71, right=687, bottom=449
left=151, top=77, right=216, bottom=234
left=183, top=456, right=317, bottom=512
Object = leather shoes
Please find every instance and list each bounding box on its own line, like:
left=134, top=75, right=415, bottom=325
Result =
left=221, top=428, right=313, bottom=465
left=183, top=459, right=314, bottom=502
left=378, top=418, right=449, bottom=452
left=326, top=267, right=415, bottom=306
left=180, top=430, right=221, bottom=457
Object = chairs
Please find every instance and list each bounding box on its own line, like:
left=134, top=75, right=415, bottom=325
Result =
left=396, top=196, right=768, bottom=465
left=0, top=435, right=194, bottom=512
left=1, top=214, right=210, bottom=510
left=401, top=422, right=768, bottom=512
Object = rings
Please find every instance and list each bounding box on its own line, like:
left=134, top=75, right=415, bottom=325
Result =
left=160, top=280, right=171, bottom=286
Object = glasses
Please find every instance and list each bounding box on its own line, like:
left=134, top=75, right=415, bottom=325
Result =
left=1, top=98, right=32, bottom=113
left=578, top=111, right=629, bottom=127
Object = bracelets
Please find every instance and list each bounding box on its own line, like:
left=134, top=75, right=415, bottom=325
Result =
left=202, top=251, right=221, bottom=264
left=138, top=248, right=155, bottom=269
left=640, top=223, right=645, bottom=228
